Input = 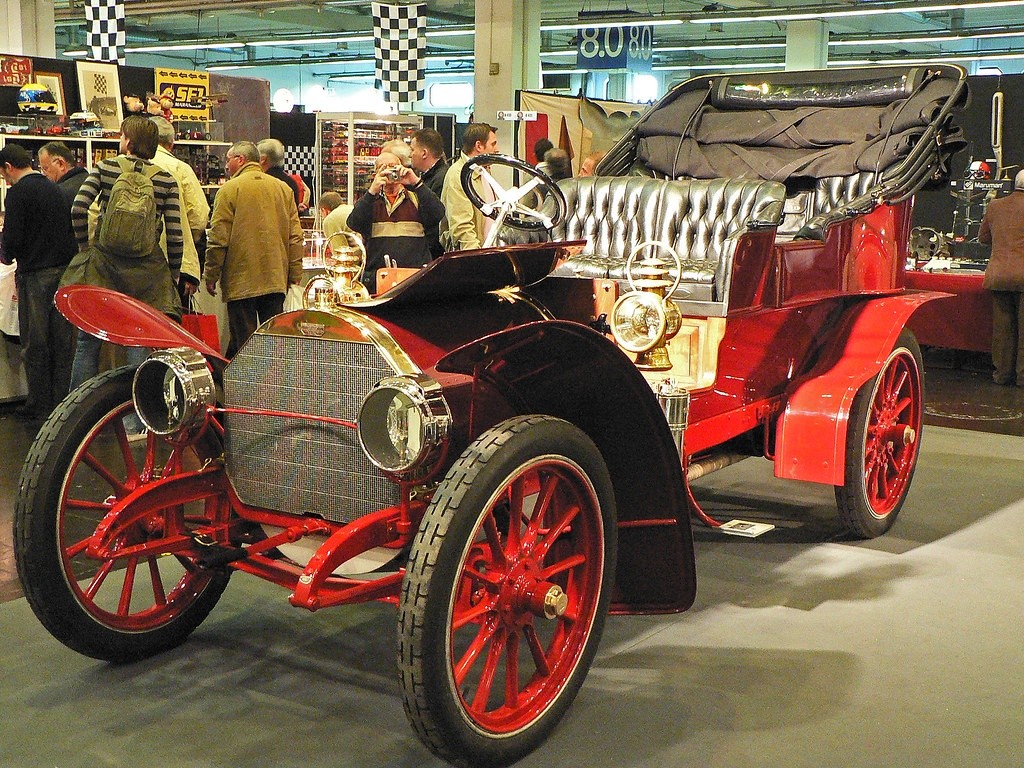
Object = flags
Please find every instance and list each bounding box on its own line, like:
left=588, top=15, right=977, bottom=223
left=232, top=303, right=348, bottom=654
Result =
left=85, top=0, right=126, bottom=67
left=370, top=2, right=427, bottom=103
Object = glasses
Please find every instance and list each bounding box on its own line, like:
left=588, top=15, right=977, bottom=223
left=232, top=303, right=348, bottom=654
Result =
left=963, top=170, right=985, bottom=179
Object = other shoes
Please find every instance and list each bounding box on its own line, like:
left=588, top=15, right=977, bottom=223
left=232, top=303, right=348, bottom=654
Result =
left=16, top=406, right=42, bottom=417
left=126, top=428, right=148, bottom=442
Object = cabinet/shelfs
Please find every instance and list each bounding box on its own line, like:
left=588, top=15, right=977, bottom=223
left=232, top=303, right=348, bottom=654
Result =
left=0, top=131, right=234, bottom=213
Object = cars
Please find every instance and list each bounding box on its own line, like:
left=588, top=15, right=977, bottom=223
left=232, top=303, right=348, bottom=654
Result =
left=9, top=62, right=973, bottom=767
left=0, top=122, right=31, bottom=134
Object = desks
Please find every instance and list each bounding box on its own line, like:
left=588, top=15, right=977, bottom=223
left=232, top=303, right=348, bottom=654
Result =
left=904, top=262, right=991, bottom=370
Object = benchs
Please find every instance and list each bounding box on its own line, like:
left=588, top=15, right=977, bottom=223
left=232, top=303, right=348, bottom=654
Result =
left=627, top=164, right=877, bottom=242
left=488, top=175, right=789, bottom=313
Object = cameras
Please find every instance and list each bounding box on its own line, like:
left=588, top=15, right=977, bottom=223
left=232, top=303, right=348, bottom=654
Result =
left=384, top=169, right=402, bottom=182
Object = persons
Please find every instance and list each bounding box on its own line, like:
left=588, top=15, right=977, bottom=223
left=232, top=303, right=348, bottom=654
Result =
left=520, top=139, right=573, bottom=213
left=439, top=124, right=506, bottom=251
left=577, top=152, right=603, bottom=177
left=382, top=129, right=448, bottom=260
left=318, top=193, right=361, bottom=256
left=37, top=142, right=90, bottom=198
left=203, top=142, right=309, bottom=359
left=88, top=113, right=210, bottom=310
left=70, top=115, right=183, bottom=442
left=0, top=145, right=74, bottom=418
left=979, top=168, right=1024, bottom=387
left=348, top=153, right=446, bottom=294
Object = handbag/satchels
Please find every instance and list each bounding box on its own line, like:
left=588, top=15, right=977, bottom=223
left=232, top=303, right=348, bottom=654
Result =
left=183, top=293, right=221, bottom=372
left=283, top=286, right=308, bottom=314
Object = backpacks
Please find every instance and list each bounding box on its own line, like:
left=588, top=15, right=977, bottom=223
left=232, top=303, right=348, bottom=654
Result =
left=99, top=156, right=164, bottom=257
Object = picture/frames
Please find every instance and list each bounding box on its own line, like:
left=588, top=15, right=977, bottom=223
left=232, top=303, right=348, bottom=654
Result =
left=75, top=58, right=125, bottom=132
left=33, top=72, right=66, bottom=116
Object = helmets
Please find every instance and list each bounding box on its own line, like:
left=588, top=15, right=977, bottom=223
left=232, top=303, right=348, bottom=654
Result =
left=969, top=161, right=991, bottom=179
